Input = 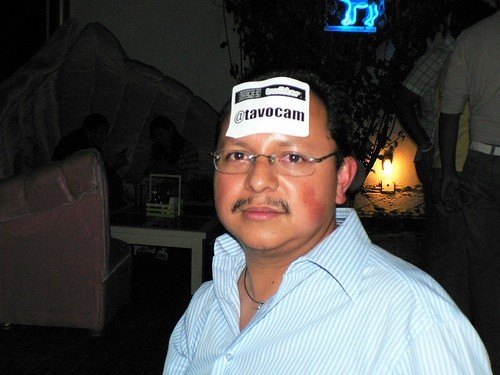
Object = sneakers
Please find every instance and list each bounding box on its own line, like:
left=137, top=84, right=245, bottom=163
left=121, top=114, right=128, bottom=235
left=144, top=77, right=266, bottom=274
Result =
left=153, top=250, right=168, bottom=260
left=137, top=246, right=156, bottom=254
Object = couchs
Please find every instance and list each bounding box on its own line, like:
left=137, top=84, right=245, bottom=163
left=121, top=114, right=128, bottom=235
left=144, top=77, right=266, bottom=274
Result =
left=0, top=20, right=219, bottom=337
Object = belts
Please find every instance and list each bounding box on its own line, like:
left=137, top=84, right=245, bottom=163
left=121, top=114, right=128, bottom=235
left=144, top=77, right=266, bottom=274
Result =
left=469, top=142, right=500, bottom=157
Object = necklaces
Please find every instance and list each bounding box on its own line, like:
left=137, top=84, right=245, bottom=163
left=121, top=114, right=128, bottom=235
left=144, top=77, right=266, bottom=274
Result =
left=242, top=265, right=271, bottom=306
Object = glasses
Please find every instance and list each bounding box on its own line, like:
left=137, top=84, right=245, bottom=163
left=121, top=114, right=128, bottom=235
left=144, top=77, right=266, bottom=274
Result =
left=209, top=150, right=342, bottom=176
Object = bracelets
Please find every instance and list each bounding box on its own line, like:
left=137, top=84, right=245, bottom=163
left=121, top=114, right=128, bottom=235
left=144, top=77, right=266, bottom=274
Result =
left=417, top=138, right=437, bottom=154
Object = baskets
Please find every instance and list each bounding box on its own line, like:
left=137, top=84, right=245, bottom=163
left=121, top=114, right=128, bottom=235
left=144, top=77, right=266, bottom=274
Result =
left=145, top=174, right=184, bottom=220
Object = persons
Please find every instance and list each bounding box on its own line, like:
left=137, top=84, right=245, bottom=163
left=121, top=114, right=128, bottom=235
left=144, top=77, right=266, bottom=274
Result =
left=53, top=110, right=204, bottom=217
left=160, top=71, right=495, bottom=375
left=436, top=0, right=500, bottom=333
left=393, top=0, right=460, bottom=269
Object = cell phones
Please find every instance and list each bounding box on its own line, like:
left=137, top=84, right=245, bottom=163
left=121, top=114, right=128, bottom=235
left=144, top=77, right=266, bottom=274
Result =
left=113, top=148, right=128, bottom=158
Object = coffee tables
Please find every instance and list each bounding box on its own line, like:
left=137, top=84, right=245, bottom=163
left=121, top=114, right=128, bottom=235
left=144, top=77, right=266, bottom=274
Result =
left=110, top=206, right=221, bottom=295
